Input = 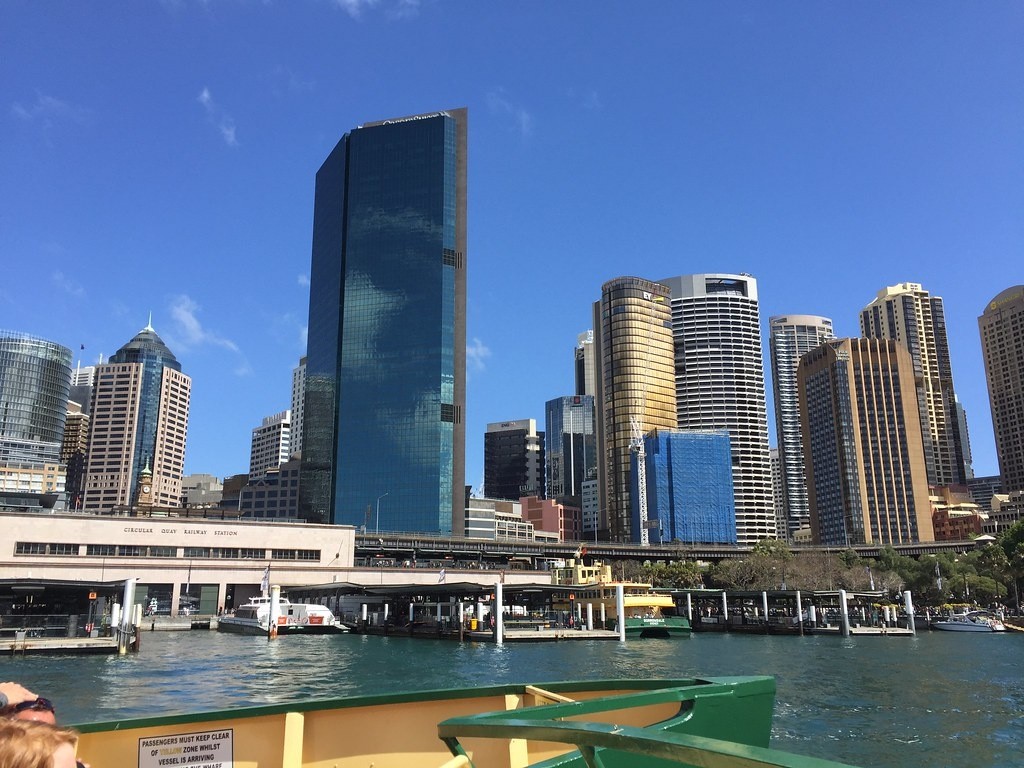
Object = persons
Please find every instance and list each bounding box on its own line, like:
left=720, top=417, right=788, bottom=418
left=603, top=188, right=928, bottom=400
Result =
left=0, top=682, right=85, bottom=768
left=0, top=718, right=78, bottom=768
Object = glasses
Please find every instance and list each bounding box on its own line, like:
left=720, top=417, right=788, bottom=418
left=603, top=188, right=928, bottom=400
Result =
left=9, top=698, right=55, bottom=721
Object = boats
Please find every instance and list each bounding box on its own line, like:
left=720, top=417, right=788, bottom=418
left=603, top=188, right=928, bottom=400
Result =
left=930, top=609, right=1010, bottom=632
left=546, top=541, right=694, bottom=637
left=217, top=558, right=352, bottom=634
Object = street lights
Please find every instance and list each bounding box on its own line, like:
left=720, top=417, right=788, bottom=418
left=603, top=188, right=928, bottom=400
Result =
left=843, top=513, right=860, bottom=548
left=238, top=482, right=250, bottom=521
left=374, top=491, right=389, bottom=534
left=595, top=506, right=608, bottom=545
